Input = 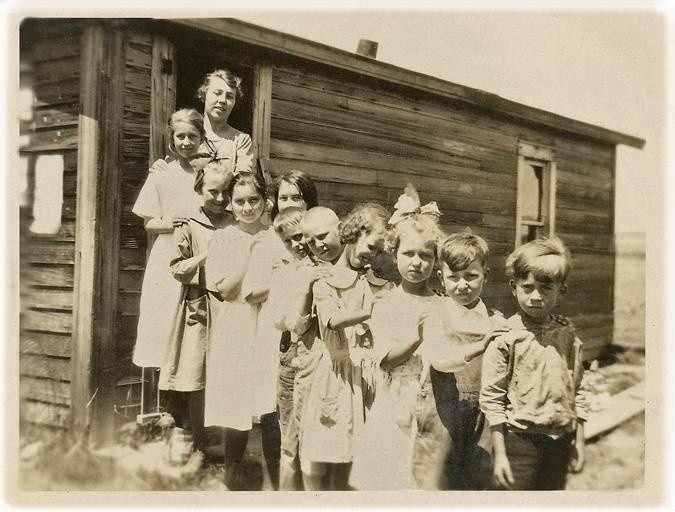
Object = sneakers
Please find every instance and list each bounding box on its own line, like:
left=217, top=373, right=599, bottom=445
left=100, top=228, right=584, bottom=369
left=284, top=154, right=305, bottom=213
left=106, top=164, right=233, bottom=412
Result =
left=161, top=426, right=194, bottom=466
